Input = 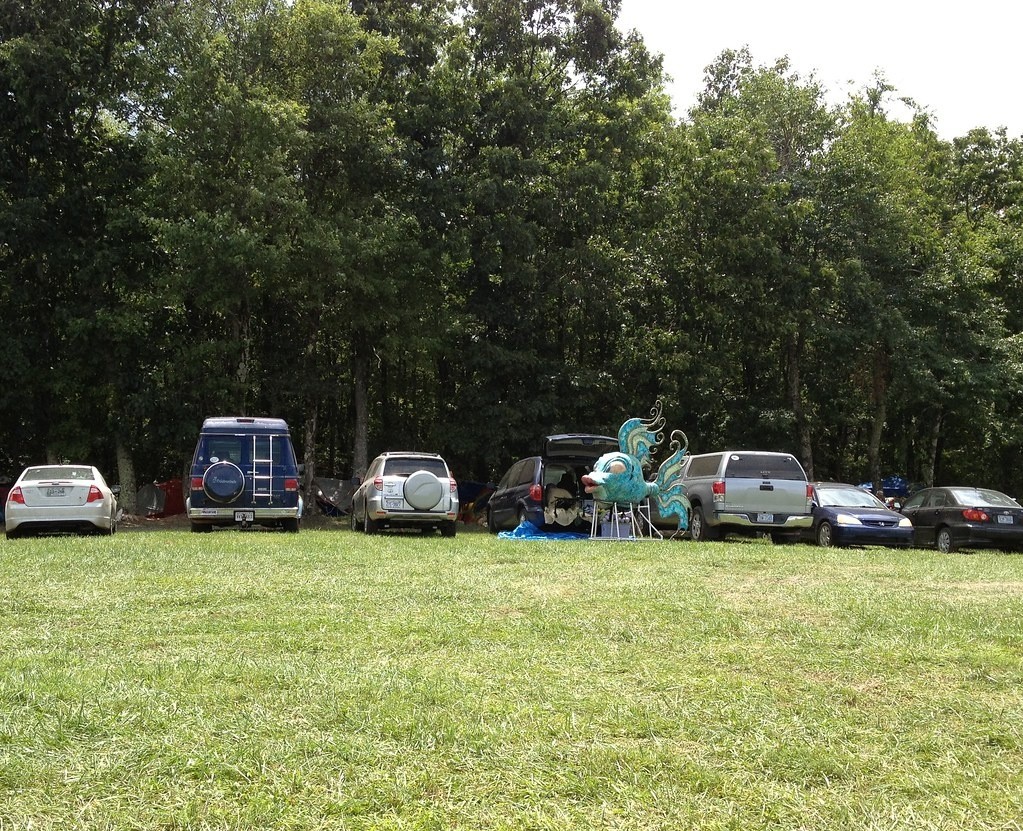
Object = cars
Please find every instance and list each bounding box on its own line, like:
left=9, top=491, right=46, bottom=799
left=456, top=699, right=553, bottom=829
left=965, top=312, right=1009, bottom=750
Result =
left=804, top=481, right=914, bottom=549
left=856, top=477, right=913, bottom=499
left=5, top=465, right=122, bottom=540
left=896, top=486, right=1023, bottom=554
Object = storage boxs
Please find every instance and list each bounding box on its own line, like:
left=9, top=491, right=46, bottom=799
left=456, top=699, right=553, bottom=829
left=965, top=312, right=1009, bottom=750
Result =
left=601, top=522, right=629, bottom=537
left=584, top=500, right=594, bottom=507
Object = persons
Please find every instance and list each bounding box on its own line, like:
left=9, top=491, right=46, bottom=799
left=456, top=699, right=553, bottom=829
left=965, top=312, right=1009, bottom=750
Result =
left=876, top=491, right=904, bottom=512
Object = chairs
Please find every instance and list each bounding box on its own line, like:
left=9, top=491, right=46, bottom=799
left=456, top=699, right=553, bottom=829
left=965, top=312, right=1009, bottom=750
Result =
left=935, top=499, right=947, bottom=506
left=216, top=451, right=233, bottom=462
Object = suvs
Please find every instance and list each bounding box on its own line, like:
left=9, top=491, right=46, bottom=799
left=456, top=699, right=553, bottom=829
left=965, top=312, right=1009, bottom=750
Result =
left=187, top=416, right=307, bottom=534
left=352, top=451, right=460, bottom=540
left=639, top=452, right=815, bottom=540
left=488, top=433, right=622, bottom=536
